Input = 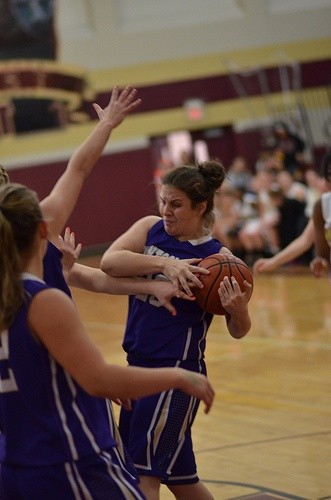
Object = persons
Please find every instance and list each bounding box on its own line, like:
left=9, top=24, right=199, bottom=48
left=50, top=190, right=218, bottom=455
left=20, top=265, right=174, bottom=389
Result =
left=101, top=161, right=251, bottom=500
left=213, top=122, right=331, bottom=278
left=0, top=183, right=215, bottom=500
left=0, top=85, right=142, bottom=300
left=59, top=225, right=199, bottom=315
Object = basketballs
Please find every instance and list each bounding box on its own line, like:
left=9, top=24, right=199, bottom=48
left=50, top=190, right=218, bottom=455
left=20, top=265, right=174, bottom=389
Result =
left=191, top=252, right=253, bottom=316
